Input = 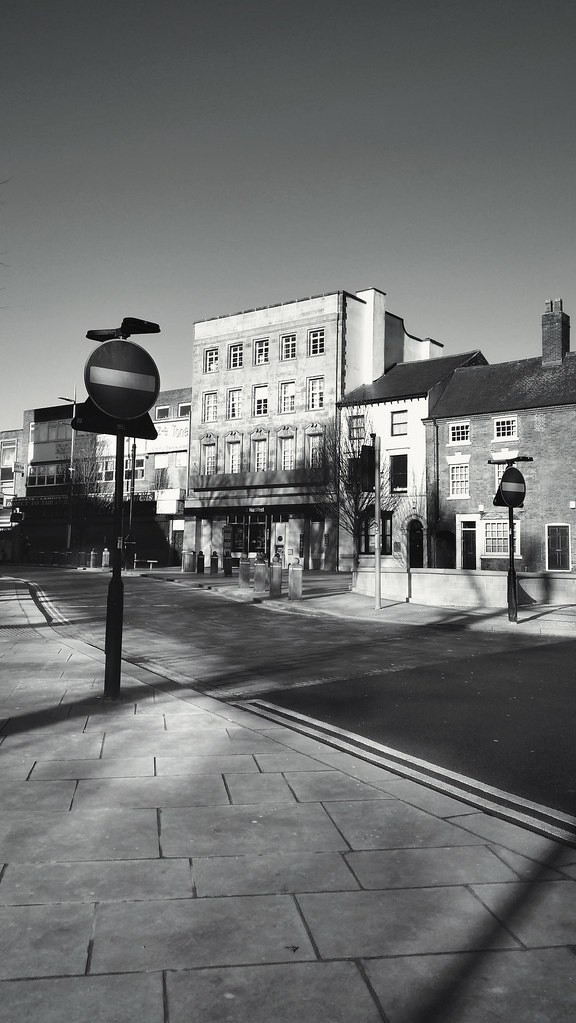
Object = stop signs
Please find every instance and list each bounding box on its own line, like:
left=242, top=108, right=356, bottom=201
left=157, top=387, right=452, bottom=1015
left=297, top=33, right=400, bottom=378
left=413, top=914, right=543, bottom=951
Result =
left=500, top=468, right=526, bottom=508
left=83, top=339, right=161, bottom=421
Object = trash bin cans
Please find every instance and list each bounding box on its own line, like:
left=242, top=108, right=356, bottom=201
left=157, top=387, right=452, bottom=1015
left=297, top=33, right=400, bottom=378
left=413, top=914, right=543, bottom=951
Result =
left=181, top=551, right=196, bottom=572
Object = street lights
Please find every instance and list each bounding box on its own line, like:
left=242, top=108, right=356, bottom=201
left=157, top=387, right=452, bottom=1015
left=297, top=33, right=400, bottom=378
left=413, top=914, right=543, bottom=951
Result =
left=58, top=397, right=76, bottom=551
left=488, top=456, right=534, bottom=623
left=87, top=319, right=161, bottom=698
